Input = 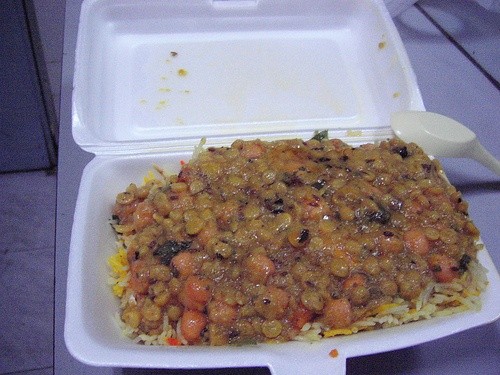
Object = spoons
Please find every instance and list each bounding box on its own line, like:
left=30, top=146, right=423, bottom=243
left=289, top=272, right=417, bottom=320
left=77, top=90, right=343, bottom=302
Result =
left=392, top=110, right=500, bottom=177
